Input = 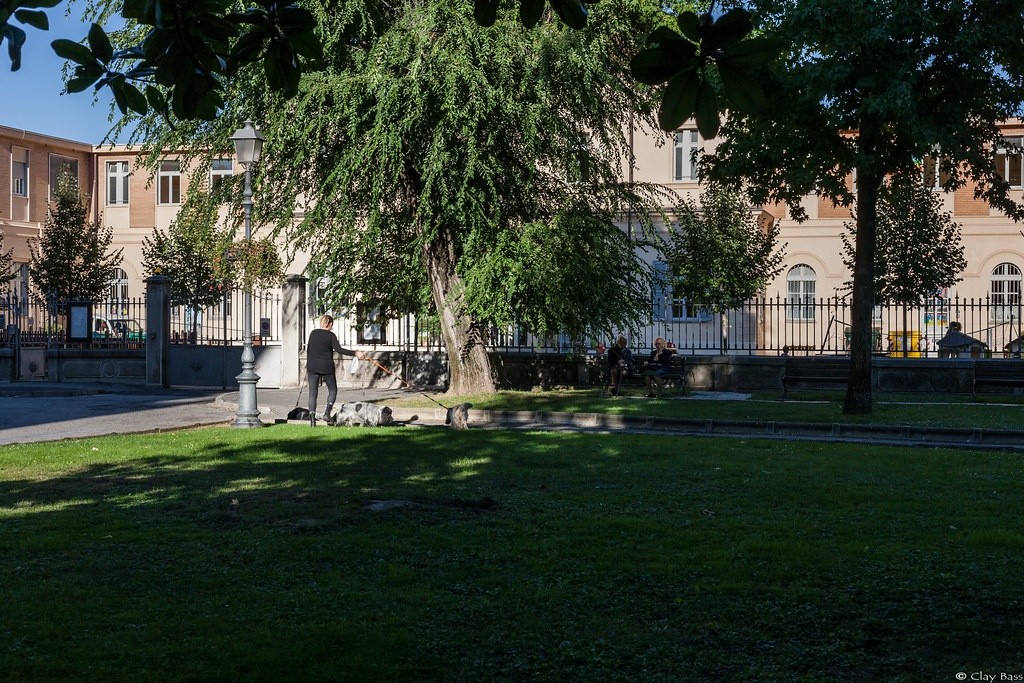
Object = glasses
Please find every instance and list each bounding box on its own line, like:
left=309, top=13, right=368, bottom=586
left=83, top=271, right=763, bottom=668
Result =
left=653, top=342, right=662, bottom=345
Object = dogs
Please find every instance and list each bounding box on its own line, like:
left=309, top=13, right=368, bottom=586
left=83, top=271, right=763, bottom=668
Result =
left=323, top=402, right=394, bottom=427
left=444, top=402, right=473, bottom=430
left=287, top=407, right=318, bottom=420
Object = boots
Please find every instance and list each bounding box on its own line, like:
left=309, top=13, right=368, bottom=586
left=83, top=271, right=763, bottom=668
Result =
left=323, top=404, right=334, bottom=425
left=310, top=412, right=316, bottom=428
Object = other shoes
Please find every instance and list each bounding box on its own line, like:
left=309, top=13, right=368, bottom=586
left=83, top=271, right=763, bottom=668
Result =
left=656, top=389, right=664, bottom=396
left=645, top=390, right=654, bottom=397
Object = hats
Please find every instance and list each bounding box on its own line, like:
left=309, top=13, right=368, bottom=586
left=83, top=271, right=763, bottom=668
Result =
left=617, top=335, right=626, bottom=345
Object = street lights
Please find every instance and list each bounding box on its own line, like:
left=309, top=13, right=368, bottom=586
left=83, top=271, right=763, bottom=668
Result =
left=228, top=117, right=267, bottom=432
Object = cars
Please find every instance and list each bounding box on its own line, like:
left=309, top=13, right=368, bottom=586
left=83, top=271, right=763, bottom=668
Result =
left=91, top=313, right=147, bottom=343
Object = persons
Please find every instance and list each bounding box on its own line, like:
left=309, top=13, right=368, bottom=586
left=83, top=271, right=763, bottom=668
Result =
left=115, top=322, right=126, bottom=335
left=303, top=315, right=369, bottom=431
left=780, top=345, right=792, bottom=356
left=641, top=336, right=673, bottom=401
left=604, top=334, right=633, bottom=396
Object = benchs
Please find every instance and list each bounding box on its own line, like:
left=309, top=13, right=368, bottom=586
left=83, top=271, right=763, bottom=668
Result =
left=599, top=354, right=688, bottom=396
left=778, top=357, right=851, bottom=400
left=971, top=360, right=1024, bottom=403
